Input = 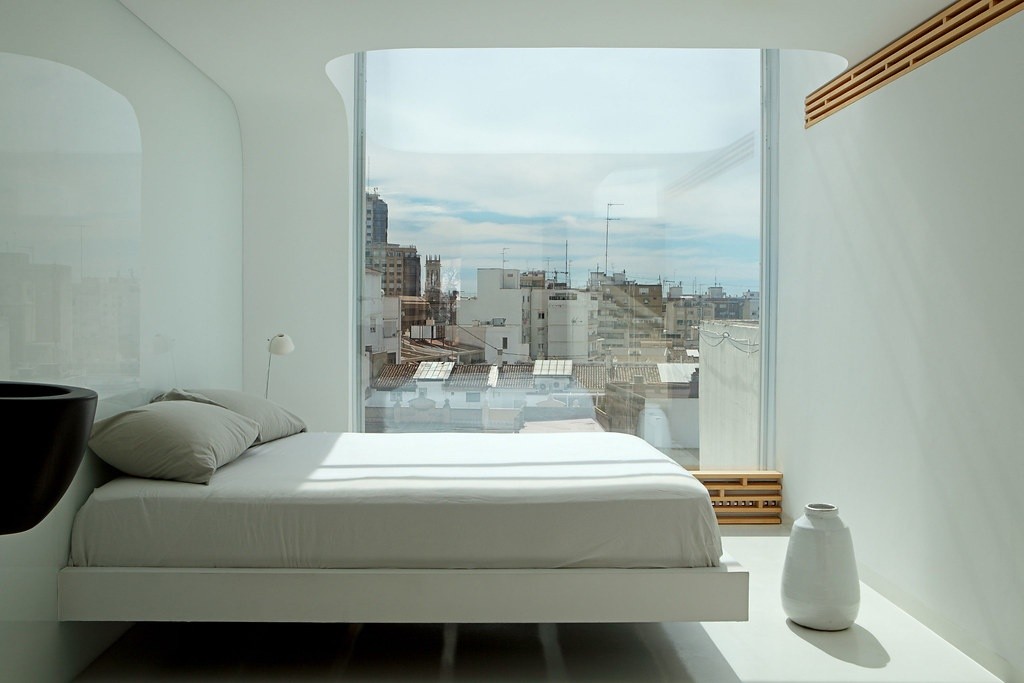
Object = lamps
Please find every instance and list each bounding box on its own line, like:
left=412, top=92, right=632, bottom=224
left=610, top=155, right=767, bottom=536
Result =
left=265, top=333, right=295, bottom=399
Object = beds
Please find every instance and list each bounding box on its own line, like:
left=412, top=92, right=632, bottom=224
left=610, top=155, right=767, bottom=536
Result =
left=57, top=431, right=751, bottom=683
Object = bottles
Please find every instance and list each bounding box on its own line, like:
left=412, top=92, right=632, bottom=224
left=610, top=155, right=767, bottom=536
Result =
left=780, top=504, right=860, bottom=631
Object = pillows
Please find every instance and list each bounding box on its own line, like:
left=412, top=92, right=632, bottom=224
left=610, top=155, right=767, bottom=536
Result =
left=156, top=393, right=308, bottom=446
left=89, top=387, right=261, bottom=487
